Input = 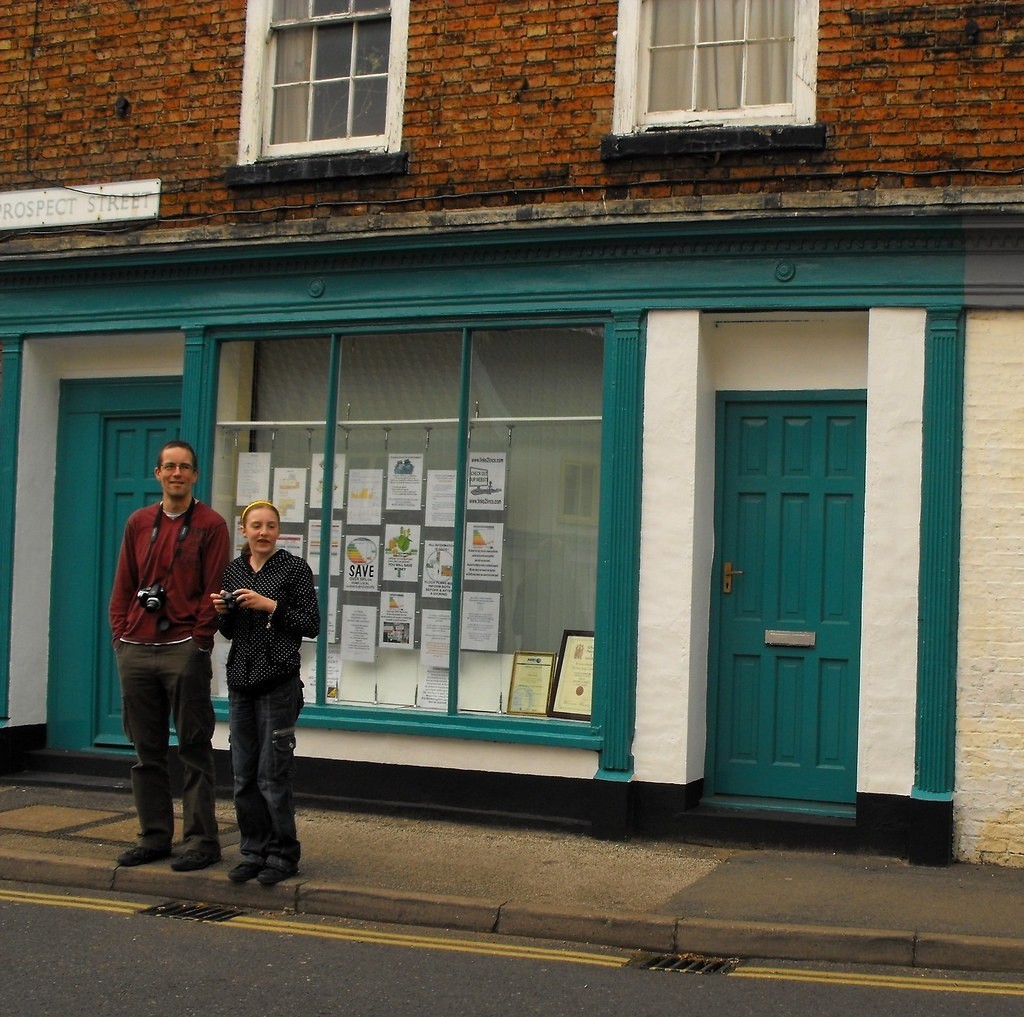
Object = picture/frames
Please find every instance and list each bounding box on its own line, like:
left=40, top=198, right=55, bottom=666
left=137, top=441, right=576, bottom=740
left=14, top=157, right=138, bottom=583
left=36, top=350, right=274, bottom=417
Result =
left=507, top=651, right=558, bottom=716
left=548, top=629, right=595, bottom=722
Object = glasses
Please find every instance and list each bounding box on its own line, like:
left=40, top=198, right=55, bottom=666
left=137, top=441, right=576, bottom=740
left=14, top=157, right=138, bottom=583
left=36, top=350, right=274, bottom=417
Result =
left=159, top=464, right=196, bottom=473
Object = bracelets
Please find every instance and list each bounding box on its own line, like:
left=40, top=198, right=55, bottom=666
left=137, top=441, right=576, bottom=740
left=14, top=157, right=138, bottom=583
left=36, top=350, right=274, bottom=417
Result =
left=265, top=600, right=278, bottom=629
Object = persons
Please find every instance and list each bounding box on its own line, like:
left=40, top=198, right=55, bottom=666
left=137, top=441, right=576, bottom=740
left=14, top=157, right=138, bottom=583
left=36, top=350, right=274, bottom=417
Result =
left=210, top=502, right=321, bottom=884
left=109, top=440, right=231, bottom=871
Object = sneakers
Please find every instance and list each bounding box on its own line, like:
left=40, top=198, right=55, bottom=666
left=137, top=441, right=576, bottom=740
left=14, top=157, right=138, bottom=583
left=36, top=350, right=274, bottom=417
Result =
left=170, top=847, right=222, bottom=872
left=257, top=867, right=299, bottom=885
left=228, top=860, right=268, bottom=882
left=117, top=846, right=173, bottom=866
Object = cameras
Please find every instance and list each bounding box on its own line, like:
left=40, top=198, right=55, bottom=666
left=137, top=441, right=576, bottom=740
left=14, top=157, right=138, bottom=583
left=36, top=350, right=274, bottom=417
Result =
left=220, top=592, right=241, bottom=610
left=136, top=583, right=168, bottom=615
